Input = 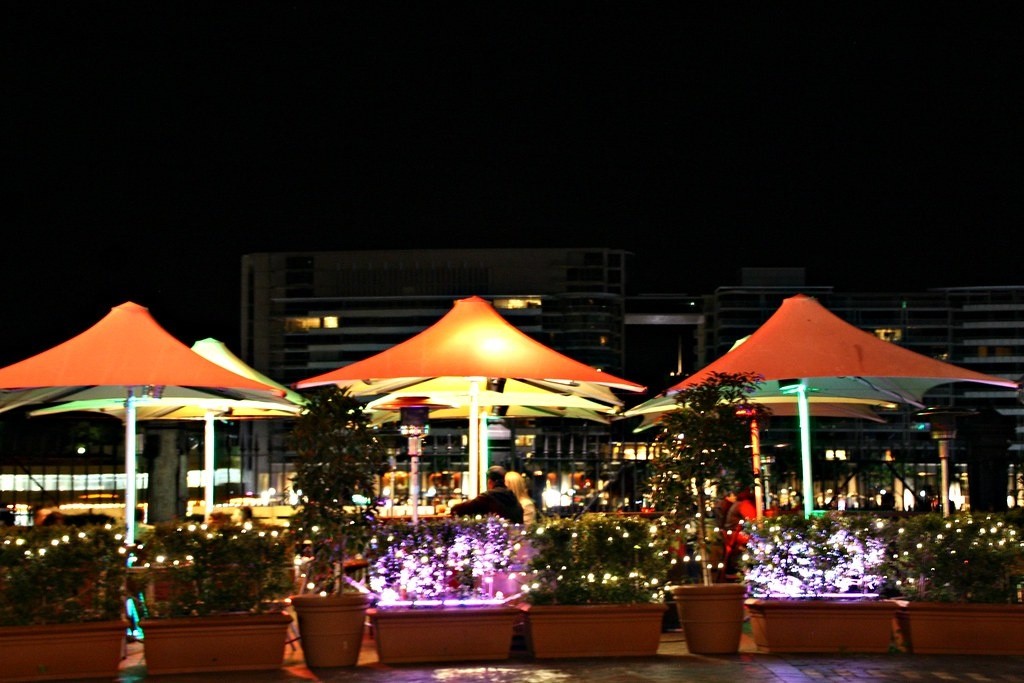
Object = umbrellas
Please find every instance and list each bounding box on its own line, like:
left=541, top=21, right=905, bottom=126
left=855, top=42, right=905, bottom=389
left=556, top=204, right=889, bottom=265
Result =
left=29, top=337, right=311, bottom=517
left=0, top=301, right=310, bottom=540
left=618, top=294, right=1018, bottom=518
left=288, top=297, right=649, bottom=496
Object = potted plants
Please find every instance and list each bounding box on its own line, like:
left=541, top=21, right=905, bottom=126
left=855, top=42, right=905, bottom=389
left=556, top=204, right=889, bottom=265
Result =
left=1, top=371, right=1024, bottom=683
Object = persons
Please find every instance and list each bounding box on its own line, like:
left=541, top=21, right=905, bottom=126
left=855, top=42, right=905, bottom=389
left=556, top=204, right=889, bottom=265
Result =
left=0, top=511, right=15, bottom=526
left=450, top=465, right=524, bottom=524
left=208, top=512, right=226, bottom=528
left=504, top=472, right=536, bottom=523
left=135, top=505, right=154, bottom=539
left=726, top=485, right=757, bottom=523
left=33, top=507, right=52, bottom=525
left=231, top=506, right=252, bottom=526
left=717, top=487, right=736, bottom=515
left=42, top=512, right=65, bottom=525
